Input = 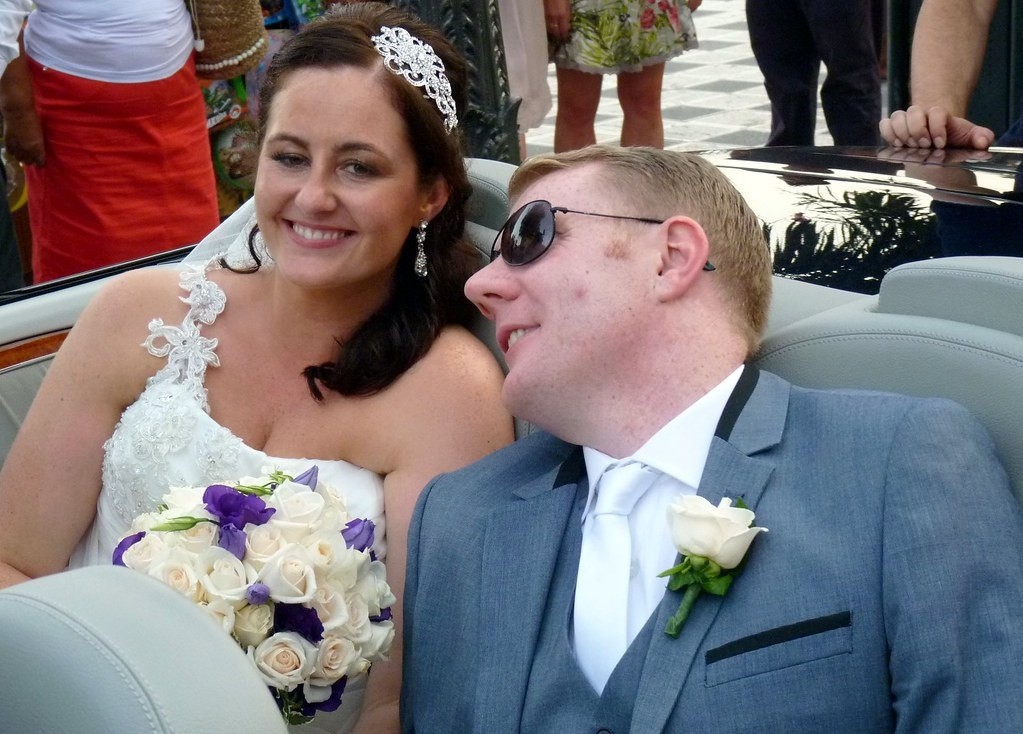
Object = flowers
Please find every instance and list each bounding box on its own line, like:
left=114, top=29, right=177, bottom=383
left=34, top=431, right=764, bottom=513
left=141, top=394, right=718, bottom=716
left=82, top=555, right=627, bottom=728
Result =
left=112, top=463, right=397, bottom=726
left=655, top=493, right=769, bottom=640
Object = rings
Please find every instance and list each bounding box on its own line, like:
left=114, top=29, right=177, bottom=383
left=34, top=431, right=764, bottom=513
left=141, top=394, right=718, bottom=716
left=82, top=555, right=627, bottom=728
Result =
left=552, top=25, right=558, bottom=27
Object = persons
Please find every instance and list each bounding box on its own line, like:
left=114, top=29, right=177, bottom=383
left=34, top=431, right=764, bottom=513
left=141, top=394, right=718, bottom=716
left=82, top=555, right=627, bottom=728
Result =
left=399, top=142, right=1022, bottom=734
left=0, top=0, right=514, bottom=734
left=746, top=0, right=1023, bottom=150
left=0, top=0, right=222, bottom=285
left=545, top=0, right=700, bottom=154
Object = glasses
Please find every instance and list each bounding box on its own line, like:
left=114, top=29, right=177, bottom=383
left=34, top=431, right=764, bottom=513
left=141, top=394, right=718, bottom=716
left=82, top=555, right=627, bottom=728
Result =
left=489, top=200, right=718, bottom=271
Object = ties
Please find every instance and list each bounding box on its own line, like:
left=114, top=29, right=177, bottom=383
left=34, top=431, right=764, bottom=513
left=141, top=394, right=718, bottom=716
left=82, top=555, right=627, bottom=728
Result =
left=572, top=463, right=662, bottom=693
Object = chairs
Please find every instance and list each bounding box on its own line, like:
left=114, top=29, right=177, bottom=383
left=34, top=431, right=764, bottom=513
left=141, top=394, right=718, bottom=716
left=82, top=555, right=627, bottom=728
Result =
left=0, top=155, right=1023, bottom=734
